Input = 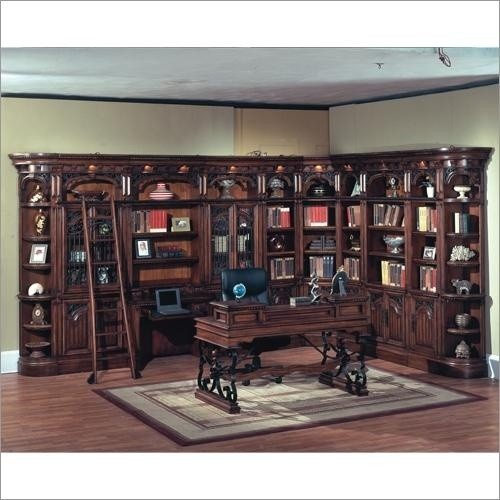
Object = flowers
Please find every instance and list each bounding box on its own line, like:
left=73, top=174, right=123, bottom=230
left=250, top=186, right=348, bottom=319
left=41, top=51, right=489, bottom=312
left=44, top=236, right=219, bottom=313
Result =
left=419, top=175, right=433, bottom=187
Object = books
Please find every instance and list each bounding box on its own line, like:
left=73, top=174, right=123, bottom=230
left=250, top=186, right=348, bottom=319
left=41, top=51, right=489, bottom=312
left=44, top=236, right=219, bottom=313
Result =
left=132, top=209, right=167, bottom=233
left=303, top=205, right=335, bottom=227
left=213, top=233, right=251, bottom=253
left=381, top=260, right=406, bottom=287
left=267, top=206, right=291, bottom=228
left=419, top=266, right=436, bottom=294
left=309, top=256, right=335, bottom=278
left=454, top=212, right=471, bottom=234
left=343, top=256, right=360, bottom=281
left=346, top=205, right=360, bottom=227
left=416, top=206, right=437, bottom=233
left=290, top=296, right=311, bottom=307
left=374, top=203, right=405, bottom=227
left=270, top=256, right=294, bottom=281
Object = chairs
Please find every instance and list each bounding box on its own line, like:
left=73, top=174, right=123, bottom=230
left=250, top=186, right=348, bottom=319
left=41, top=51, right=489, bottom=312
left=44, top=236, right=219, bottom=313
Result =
left=220, top=268, right=291, bottom=386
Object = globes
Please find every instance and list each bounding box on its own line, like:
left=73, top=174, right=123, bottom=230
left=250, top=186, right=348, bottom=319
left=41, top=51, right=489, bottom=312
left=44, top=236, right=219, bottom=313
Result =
left=233, top=283, right=246, bottom=304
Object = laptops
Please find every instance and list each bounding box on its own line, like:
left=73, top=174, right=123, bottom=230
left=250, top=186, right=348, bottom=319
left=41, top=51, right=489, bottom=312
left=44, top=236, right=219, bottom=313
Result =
left=155, top=288, right=193, bottom=316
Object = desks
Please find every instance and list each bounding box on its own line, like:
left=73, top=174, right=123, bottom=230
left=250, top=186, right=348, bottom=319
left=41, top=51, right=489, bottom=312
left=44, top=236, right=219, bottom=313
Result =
left=194, top=295, right=373, bottom=415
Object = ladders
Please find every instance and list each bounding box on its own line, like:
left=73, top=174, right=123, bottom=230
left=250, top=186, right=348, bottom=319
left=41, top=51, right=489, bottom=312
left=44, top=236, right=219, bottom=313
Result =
left=80, top=196, right=138, bottom=384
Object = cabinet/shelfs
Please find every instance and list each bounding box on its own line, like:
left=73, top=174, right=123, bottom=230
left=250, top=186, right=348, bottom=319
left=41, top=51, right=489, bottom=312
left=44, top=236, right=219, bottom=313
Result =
left=8, top=147, right=495, bottom=379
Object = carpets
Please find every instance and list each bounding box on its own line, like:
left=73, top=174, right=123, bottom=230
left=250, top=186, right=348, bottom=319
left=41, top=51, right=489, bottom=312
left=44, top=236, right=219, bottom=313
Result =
left=92, top=361, right=489, bottom=447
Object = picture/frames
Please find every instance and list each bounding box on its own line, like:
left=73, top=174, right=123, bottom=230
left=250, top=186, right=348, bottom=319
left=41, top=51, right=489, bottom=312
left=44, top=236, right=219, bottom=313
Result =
left=135, top=239, right=152, bottom=259
left=29, top=243, right=48, bottom=264
left=423, top=245, right=437, bottom=260
left=171, top=216, right=191, bottom=232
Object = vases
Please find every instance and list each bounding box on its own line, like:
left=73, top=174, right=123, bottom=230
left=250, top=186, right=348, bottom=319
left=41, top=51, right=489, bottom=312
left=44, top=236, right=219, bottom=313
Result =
left=427, top=187, right=435, bottom=198
